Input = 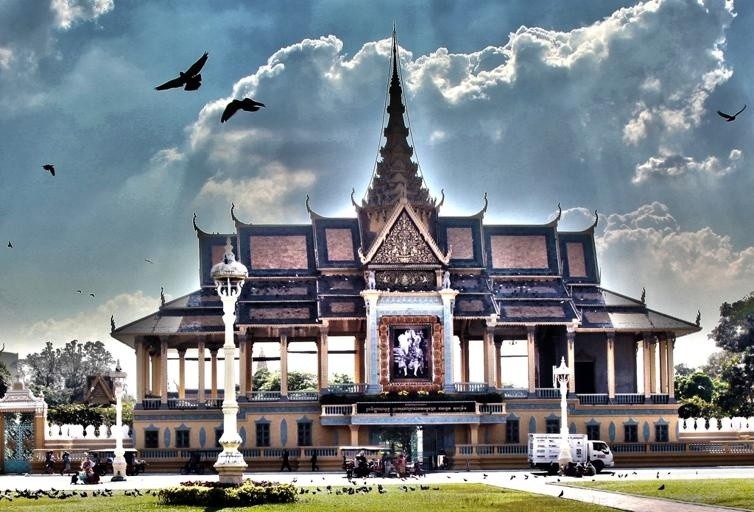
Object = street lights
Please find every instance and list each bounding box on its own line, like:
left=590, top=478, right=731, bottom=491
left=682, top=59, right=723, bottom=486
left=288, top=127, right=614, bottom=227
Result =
left=553, top=355, right=572, bottom=473
left=109, top=359, right=127, bottom=480
left=210, top=237, right=249, bottom=485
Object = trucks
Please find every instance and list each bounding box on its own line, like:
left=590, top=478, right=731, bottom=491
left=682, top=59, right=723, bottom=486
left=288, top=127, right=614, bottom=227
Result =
left=528, top=433, right=614, bottom=474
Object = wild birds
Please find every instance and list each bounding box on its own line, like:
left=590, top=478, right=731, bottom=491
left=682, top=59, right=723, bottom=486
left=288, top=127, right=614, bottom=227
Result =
left=41, top=164, right=56, bottom=176
left=658, top=484, right=665, bottom=491
left=8, top=241, right=13, bottom=247
left=717, top=104, right=747, bottom=122
left=0, top=487, right=156, bottom=501
left=221, top=98, right=266, bottom=123
left=299, top=484, right=440, bottom=496
left=154, top=51, right=209, bottom=91
left=558, top=489, right=565, bottom=497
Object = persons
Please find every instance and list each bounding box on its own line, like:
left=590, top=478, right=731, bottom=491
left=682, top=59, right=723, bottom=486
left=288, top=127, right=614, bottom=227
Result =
left=41, top=448, right=102, bottom=485
left=582, top=461, right=596, bottom=476
left=309, top=448, right=319, bottom=471
left=351, top=447, right=424, bottom=480
left=572, top=462, right=583, bottom=478
left=278, top=448, right=291, bottom=472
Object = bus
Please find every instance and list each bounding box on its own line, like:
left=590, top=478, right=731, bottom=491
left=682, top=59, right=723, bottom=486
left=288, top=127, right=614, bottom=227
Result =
left=87, top=449, right=146, bottom=476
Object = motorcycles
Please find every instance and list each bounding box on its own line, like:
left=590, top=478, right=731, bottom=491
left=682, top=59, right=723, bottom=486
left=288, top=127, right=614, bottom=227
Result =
left=180, top=460, right=204, bottom=475
